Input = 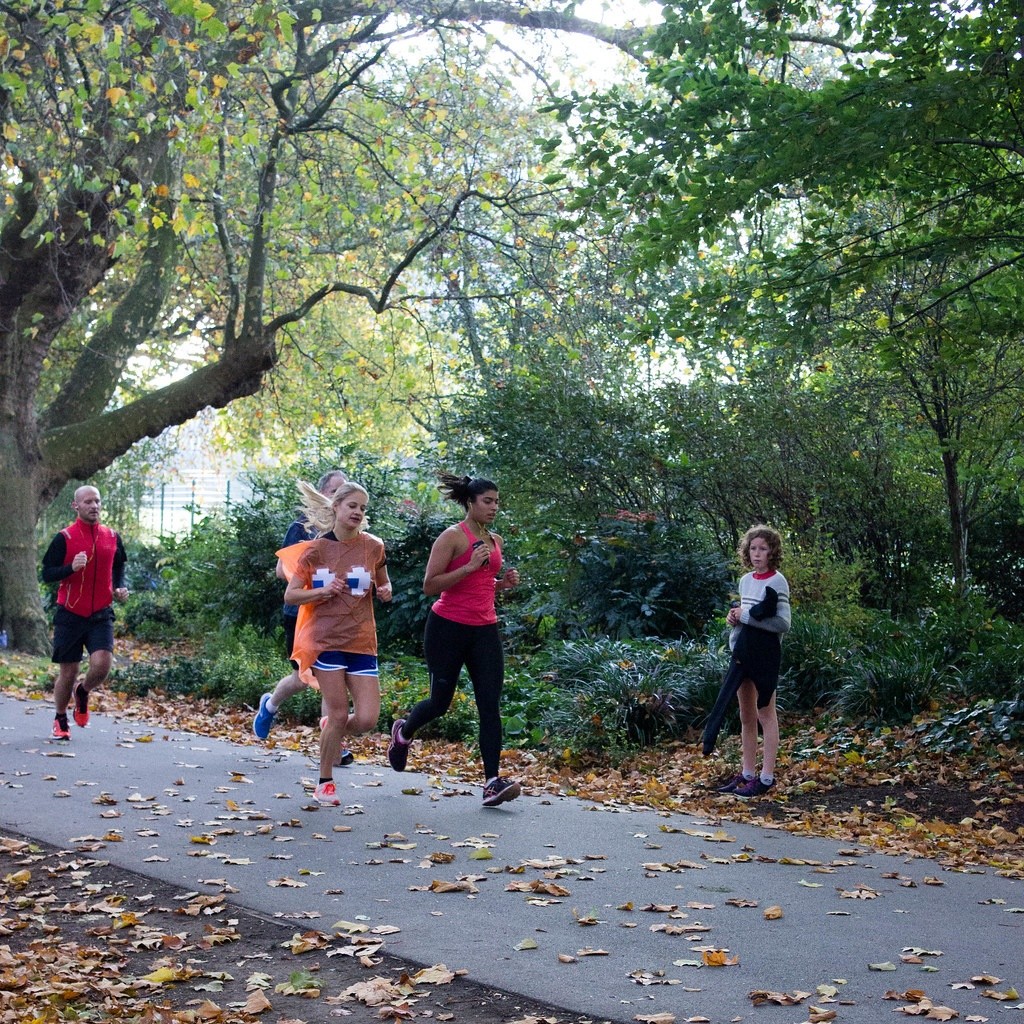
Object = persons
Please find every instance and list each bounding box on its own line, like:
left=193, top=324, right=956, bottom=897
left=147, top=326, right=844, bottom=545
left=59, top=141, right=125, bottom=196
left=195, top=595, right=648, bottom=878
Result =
left=386, top=475, right=523, bottom=807
left=716, top=526, right=791, bottom=799
left=283, top=480, right=392, bottom=807
left=40, top=485, right=129, bottom=742
left=252, top=472, right=355, bottom=764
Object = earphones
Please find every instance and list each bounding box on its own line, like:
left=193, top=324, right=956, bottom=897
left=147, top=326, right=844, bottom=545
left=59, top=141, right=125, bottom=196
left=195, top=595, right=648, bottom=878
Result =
left=75, top=507, right=79, bottom=510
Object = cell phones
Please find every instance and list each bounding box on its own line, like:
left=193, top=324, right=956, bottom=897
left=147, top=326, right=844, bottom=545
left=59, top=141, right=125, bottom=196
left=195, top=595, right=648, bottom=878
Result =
left=473, top=540, right=490, bottom=566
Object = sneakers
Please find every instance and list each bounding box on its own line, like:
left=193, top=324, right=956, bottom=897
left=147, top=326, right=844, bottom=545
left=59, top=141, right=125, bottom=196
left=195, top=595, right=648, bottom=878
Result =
left=321, top=715, right=348, bottom=754
left=717, top=771, right=759, bottom=796
left=252, top=693, right=278, bottom=739
left=340, top=747, right=354, bottom=766
left=72, top=681, right=89, bottom=728
left=314, top=781, right=341, bottom=807
left=734, top=777, right=777, bottom=799
left=387, top=719, right=412, bottom=772
left=52, top=712, right=72, bottom=741
left=482, top=777, right=520, bottom=807
